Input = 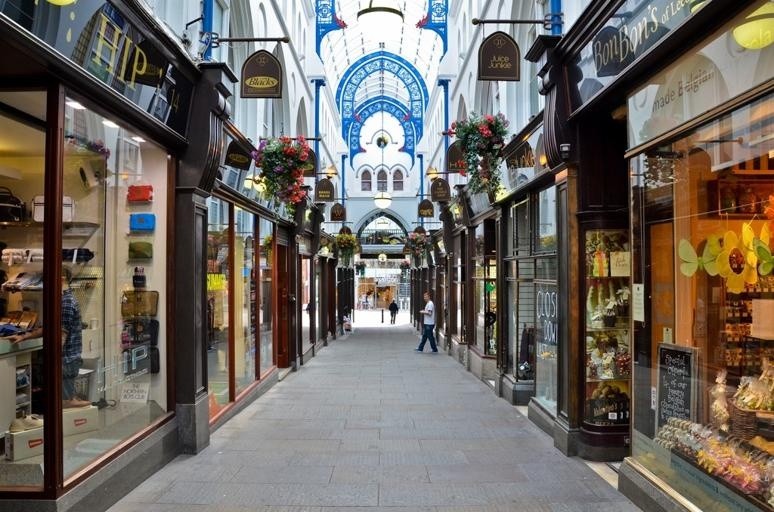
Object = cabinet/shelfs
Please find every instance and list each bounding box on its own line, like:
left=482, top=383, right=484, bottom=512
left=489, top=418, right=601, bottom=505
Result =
left=2, top=222, right=99, bottom=444
left=720, top=261, right=774, bottom=381
left=706, top=178, right=774, bottom=220
left=581, top=272, right=631, bottom=445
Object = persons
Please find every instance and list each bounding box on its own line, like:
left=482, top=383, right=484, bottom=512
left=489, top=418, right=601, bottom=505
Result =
left=1, top=265, right=82, bottom=401
left=414, top=292, right=438, bottom=353
left=389, top=298, right=398, bottom=325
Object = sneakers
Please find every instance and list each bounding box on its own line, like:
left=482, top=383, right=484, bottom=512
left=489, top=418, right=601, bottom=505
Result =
left=9, top=415, right=45, bottom=433
left=62, top=398, right=92, bottom=409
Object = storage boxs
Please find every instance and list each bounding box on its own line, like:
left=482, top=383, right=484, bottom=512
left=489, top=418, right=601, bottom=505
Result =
left=5, top=428, right=47, bottom=460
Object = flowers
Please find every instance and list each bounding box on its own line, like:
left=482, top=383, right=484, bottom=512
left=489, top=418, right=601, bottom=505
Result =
left=399, top=231, right=430, bottom=258
left=449, top=111, right=511, bottom=191
left=334, top=232, right=355, bottom=266
left=248, top=134, right=310, bottom=210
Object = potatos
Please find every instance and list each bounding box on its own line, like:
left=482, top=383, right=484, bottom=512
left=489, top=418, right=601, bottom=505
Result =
left=591, top=382, right=629, bottom=399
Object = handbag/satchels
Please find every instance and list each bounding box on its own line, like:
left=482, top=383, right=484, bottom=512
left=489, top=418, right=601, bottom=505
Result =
left=130, top=213, right=156, bottom=231
left=121, top=291, right=159, bottom=374
left=129, top=241, right=153, bottom=259
left=31, top=195, right=75, bottom=223
left=127, top=185, right=153, bottom=202
left=0, top=186, right=26, bottom=222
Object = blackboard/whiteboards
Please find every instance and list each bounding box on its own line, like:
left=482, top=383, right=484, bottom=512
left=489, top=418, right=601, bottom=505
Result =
left=654, top=341, right=699, bottom=439
